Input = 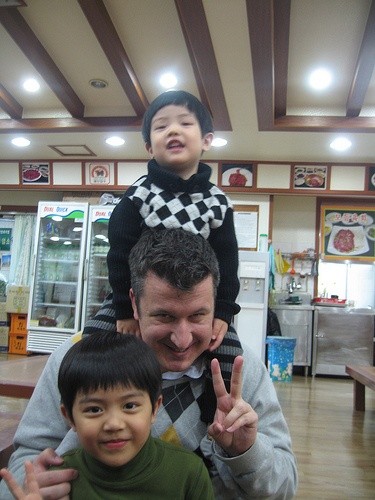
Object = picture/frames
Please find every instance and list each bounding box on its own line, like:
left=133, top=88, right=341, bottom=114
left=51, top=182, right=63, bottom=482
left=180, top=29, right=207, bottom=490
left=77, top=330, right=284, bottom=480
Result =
left=365, top=165, right=375, bottom=193
left=289, top=163, right=331, bottom=193
left=19, top=161, right=53, bottom=185
left=320, top=206, right=375, bottom=261
left=82, top=160, right=118, bottom=187
left=217, top=160, right=258, bottom=190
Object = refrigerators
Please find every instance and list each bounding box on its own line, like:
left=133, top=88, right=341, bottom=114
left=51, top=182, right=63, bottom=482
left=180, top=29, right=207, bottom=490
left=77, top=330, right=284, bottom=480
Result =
left=25, top=200, right=89, bottom=354
left=81, top=204, right=117, bottom=330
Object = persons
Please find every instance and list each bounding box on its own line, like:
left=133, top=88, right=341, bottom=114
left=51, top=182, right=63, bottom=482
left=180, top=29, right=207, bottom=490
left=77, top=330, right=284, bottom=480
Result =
left=0, top=331, right=215, bottom=500
left=54, top=90, right=243, bottom=492
left=0, top=228, right=297, bottom=500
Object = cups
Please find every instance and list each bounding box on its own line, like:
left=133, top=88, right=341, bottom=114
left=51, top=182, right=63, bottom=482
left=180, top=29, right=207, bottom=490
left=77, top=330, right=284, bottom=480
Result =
left=258, top=234, right=268, bottom=252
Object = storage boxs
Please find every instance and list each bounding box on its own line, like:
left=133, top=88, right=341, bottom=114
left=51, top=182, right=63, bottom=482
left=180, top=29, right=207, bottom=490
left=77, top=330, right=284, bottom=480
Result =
left=0, top=284, right=32, bottom=355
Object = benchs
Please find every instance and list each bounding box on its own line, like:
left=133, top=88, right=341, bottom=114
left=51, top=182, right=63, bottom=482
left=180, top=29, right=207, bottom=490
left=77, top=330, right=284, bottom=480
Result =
left=345, top=365, right=375, bottom=411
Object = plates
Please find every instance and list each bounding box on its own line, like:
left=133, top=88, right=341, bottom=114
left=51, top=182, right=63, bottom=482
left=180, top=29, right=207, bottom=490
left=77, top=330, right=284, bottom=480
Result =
left=222, top=168, right=253, bottom=187
left=325, top=212, right=341, bottom=224
left=22, top=168, right=42, bottom=182
left=365, top=225, right=375, bottom=241
left=39, top=166, right=48, bottom=178
left=295, top=168, right=326, bottom=187
left=326, top=225, right=370, bottom=256
left=342, top=213, right=358, bottom=225
left=92, top=166, right=108, bottom=177
left=358, top=214, right=374, bottom=225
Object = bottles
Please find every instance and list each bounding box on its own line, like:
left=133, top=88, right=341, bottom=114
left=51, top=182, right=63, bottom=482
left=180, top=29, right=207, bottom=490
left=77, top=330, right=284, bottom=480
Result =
left=269, top=287, right=275, bottom=305
